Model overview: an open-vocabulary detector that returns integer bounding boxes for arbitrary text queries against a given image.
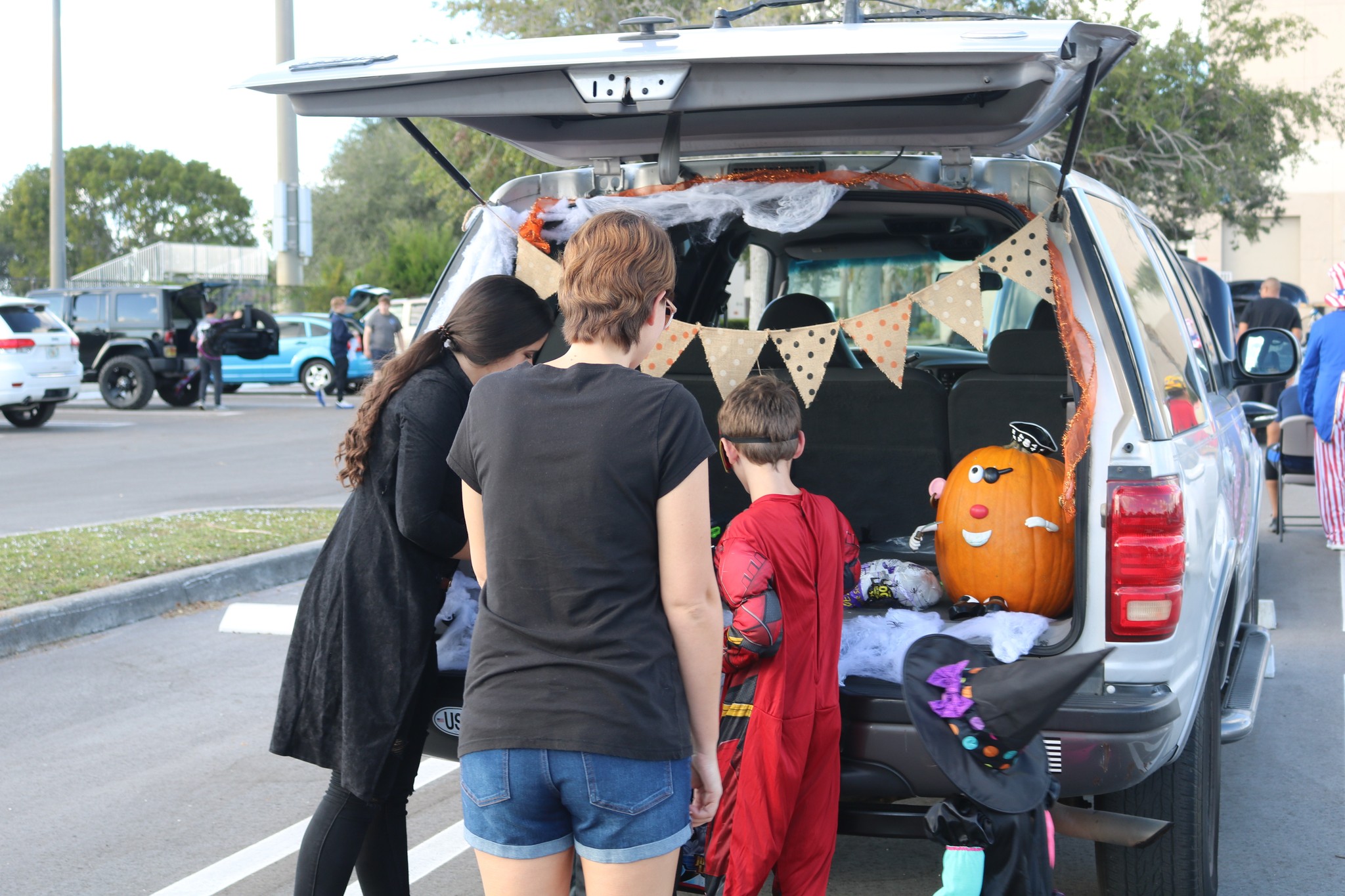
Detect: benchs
[635,335,946,543]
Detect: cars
[0,295,87,431]
[1225,280,1309,328]
[208,283,395,395]
[360,296,431,355]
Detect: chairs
[1026,298,1058,330]
[948,330,1067,469]
[753,293,863,368]
[1276,414,1323,543]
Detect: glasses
[661,297,675,326]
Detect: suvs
[240,1,1301,895]
[19,285,217,413]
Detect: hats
[902,633,1119,814]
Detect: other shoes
[336,399,353,408]
[1268,517,1285,531]
[318,387,326,406]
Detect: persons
[318,295,404,409]
[1218,413,1250,544]
[703,373,861,896]
[268,275,553,896]
[902,633,1116,896]
[1164,372,1199,433]
[446,209,724,896]
[1235,260,1345,552]
[189,303,242,410]
